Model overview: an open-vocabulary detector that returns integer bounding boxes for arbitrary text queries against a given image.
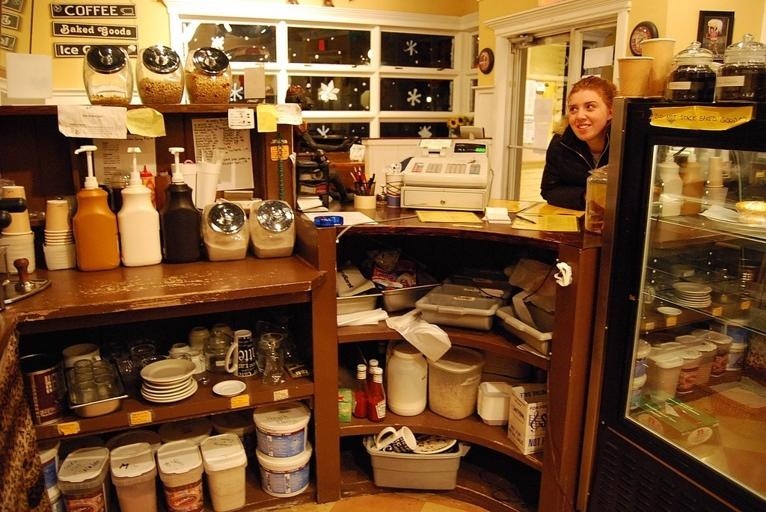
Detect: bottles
[351,356,387,422]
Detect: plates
[211,380,246,396]
[138,358,198,404]
[415,434,456,454]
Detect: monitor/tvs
[460,125,485,139]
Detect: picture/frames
[697,10,735,63]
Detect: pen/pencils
[350,165,376,196]
[516,214,537,224]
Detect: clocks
[629,21,658,56]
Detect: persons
[540,75,617,211]
[708,27,718,56]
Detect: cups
[703,148,735,208]
[638,38,676,99]
[259,333,287,385]
[374,424,418,452]
[64,343,100,367]
[72,359,119,404]
[670,280,713,309]
[43,200,77,272]
[385,173,404,207]
[119,321,231,375]
[223,330,258,378]
[0,186,36,275]
[617,54,653,96]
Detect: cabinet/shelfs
[0,103,325,512]
[296,201,597,512]
